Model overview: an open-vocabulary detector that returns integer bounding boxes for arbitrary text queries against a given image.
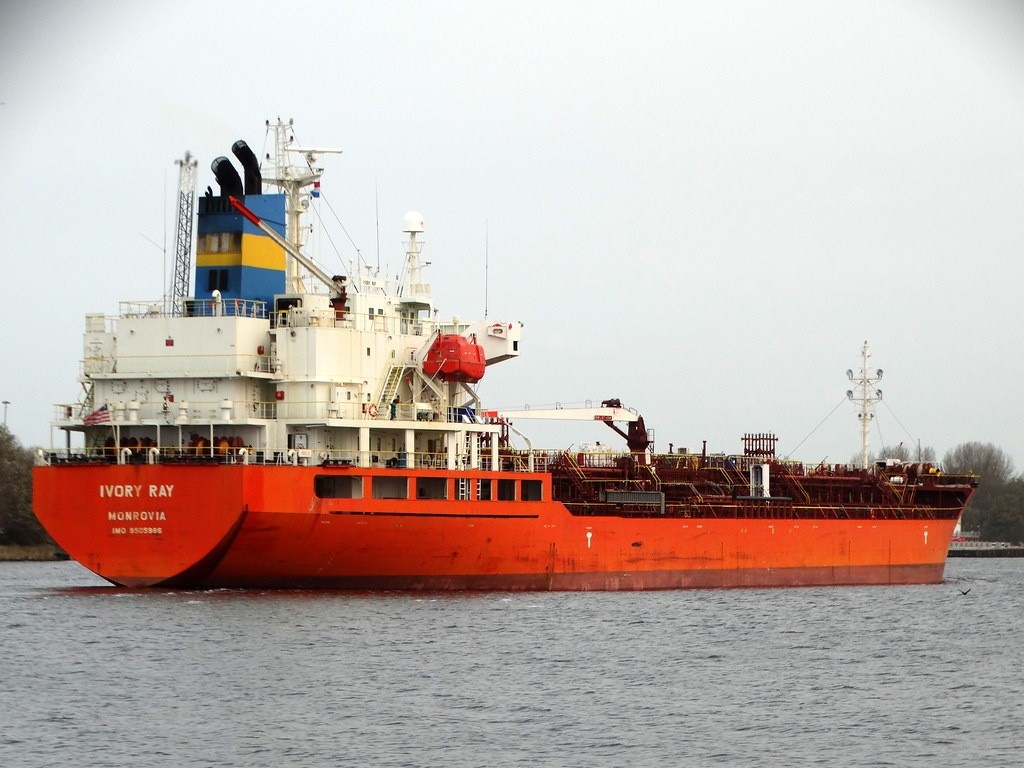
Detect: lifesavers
[369,405,378,417]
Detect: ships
[30,116,980,590]
